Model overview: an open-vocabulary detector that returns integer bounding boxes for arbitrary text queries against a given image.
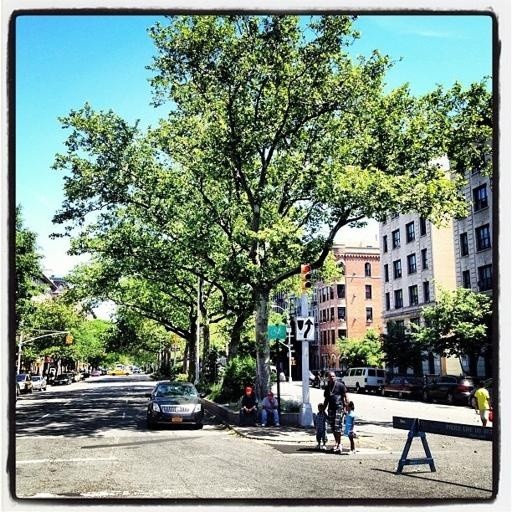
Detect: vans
[269,366,286,381]
[309,367,387,393]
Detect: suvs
[422,377,479,404]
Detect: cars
[144,381,211,430]
[391,375,421,386]
[52,369,100,385]
[107,362,140,376]
[468,378,492,408]
[16,374,47,398]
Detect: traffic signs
[170,348,180,351]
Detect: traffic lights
[300,265,311,291]
[66,335,73,344]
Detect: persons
[474,380,492,426]
[343,401,356,453]
[323,371,349,453]
[313,403,328,450]
[261,391,280,427]
[312,368,322,388]
[238,386,259,427]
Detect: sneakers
[316,445,357,455]
[239,423,282,427]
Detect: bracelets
[345,398,349,399]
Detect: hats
[245,386,252,393]
